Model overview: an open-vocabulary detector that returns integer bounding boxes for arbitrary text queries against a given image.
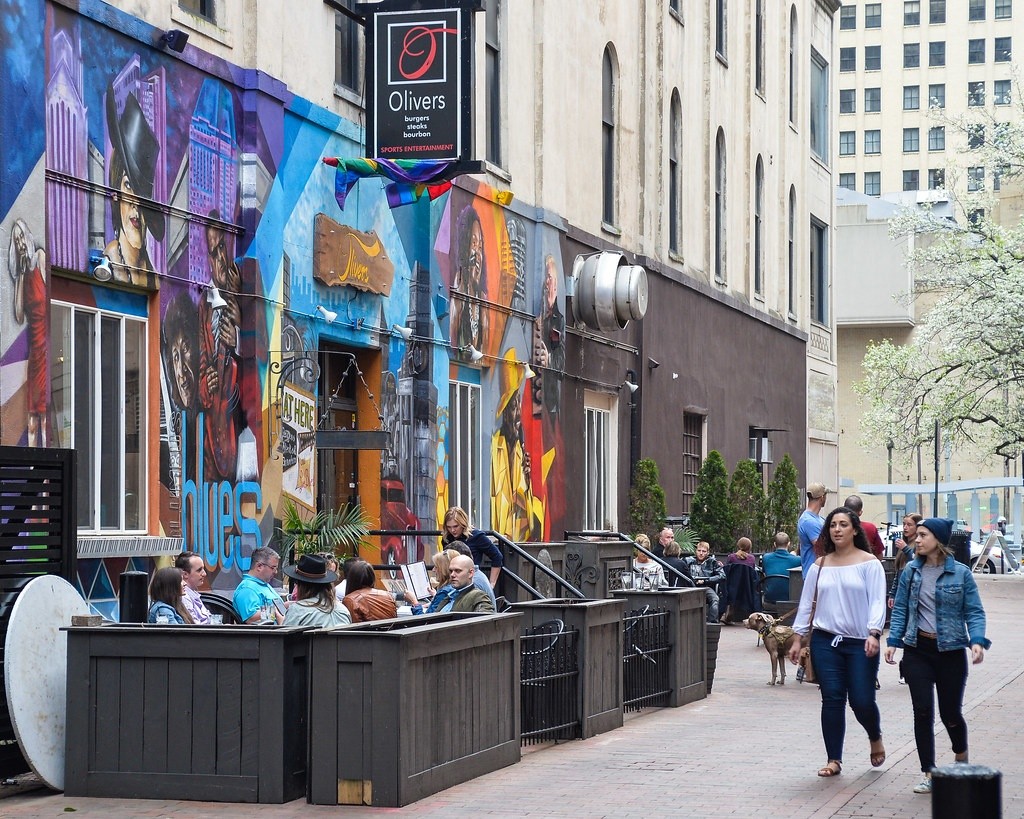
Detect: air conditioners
[748,437,774,464]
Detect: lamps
[314,304,338,321]
[392,322,414,341]
[90,256,113,280]
[518,361,537,379]
[619,379,640,394]
[164,28,190,53]
[498,190,515,206]
[462,342,483,362]
[208,288,228,311]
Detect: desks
[690,574,709,590]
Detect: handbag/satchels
[796,645,820,684]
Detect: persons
[797,484,831,684]
[844,495,884,690]
[440,506,504,590]
[789,506,888,777]
[884,518,993,793]
[888,513,924,684]
[762,532,801,603]
[147,567,195,624]
[403,540,496,615]
[174,551,214,625]
[232,546,293,626]
[335,557,360,601]
[652,528,697,587]
[342,561,398,623]
[632,534,669,587]
[275,552,352,628]
[682,541,727,622]
[727,537,755,569]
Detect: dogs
[743,612,812,686]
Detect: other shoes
[720,613,734,625]
[898,677,905,683]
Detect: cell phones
[273,598,287,616]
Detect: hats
[915,518,954,547]
[806,481,838,499]
[104,82,166,238]
[495,345,525,421]
[281,554,341,584]
[445,540,474,560]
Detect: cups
[267,605,275,622]
[690,564,698,578]
[621,572,632,591]
[210,614,223,625]
[261,605,268,625]
[634,572,645,591]
[649,573,659,592]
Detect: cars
[879,525,1019,575]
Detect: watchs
[869,632,880,640]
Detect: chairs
[725,563,758,619]
[709,570,722,595]
[620,605,650,652]
[756,575,790,647]
[519,618,564,689]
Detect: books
[399,560,433,599]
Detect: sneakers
[955,745,968,763]
[912,776,933,794]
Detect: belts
[918,630,938,639]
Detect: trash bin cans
[948,531,974,568]
[891,531,903,557]
[880,557,897,626]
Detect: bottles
[156,614,168,624]
[759,554,763,571]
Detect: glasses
[257,563,279,572]
[207,236,226,258]
[443,548,449,563]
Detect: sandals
[817,760,842,777]
[870,733,886,767]
